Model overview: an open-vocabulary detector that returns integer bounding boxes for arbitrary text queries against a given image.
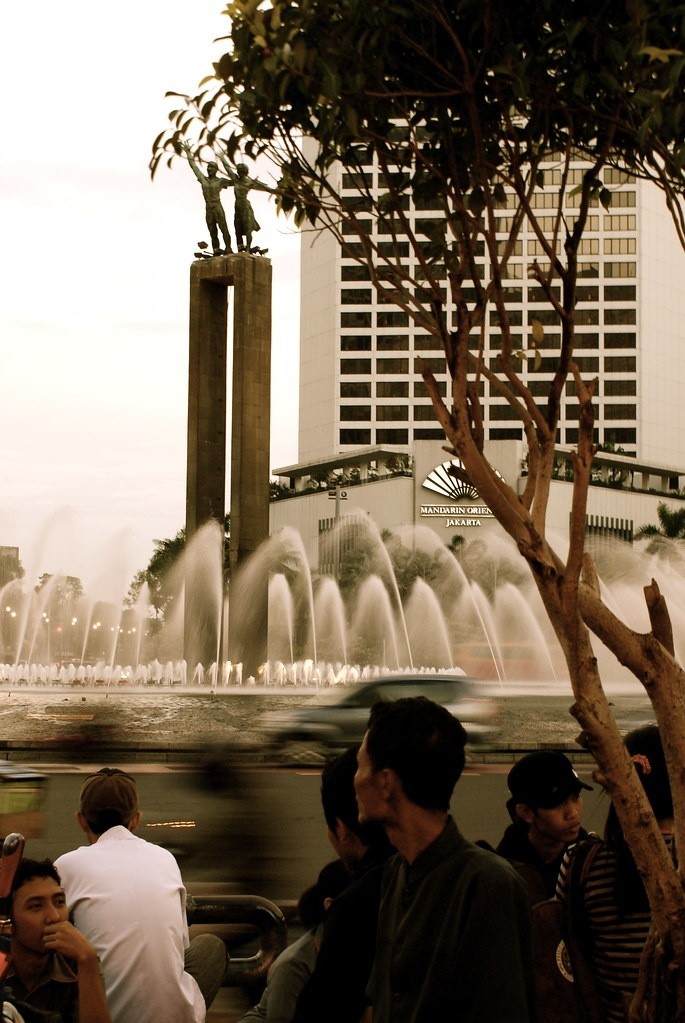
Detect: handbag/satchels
[526,841,601,1023]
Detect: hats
[79,768,138,826]
[507,751,594,809]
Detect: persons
[0,697,678,1023]
[182,140,235,256]
[216,150,276,251]
